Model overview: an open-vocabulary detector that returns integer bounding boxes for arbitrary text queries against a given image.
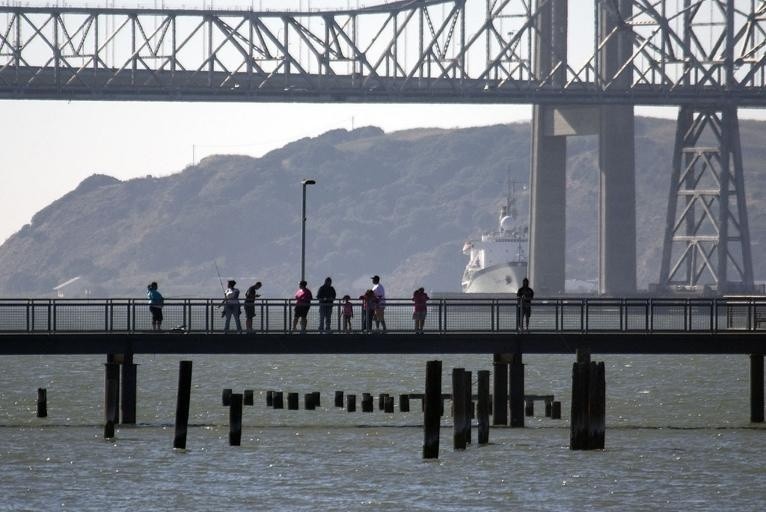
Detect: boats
[459,164,530,294]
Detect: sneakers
[373,329,387,333]
[416,330,424,334]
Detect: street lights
[298,178,318,283]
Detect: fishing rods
[215,261,229,304]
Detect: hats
[229,281,236,286]
[371,276,379,279]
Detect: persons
[411,289,430,335]
[369,274,387,333]
[146,280,164,331]
[242,281,262,334]
[418,287,428,317]
[317,276,337,335]
[515,278,534,335]
[288,281,312,335]
[359,289,380,333]
[216,279,245,334]
[338,294,354,332]
